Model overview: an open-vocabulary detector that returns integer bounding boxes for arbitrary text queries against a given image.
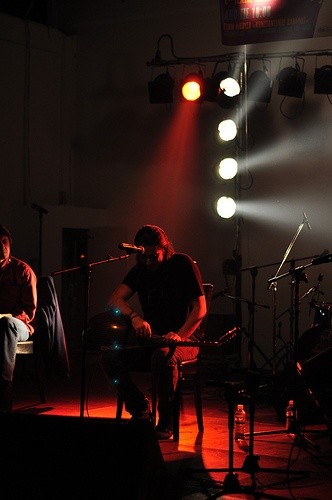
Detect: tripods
[189,218,332,500]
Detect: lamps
[148,53,332,106]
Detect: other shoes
[154,422,173,439]
[131,408,153,424]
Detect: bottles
[285,400,296,429]
[234,404,246,441]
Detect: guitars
[81,312,242,355]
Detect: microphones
[118,243,146,256]
[316,273,324,289]
[303,212,312,230]
[31,204,49,214]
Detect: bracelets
[128,310,135,317]
[130,314,139,320]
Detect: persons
[101,224,209,438]
[0,226,37,411]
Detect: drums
[312,304,332,327]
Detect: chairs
[116,283,213,442]
[15,276,70,403]
